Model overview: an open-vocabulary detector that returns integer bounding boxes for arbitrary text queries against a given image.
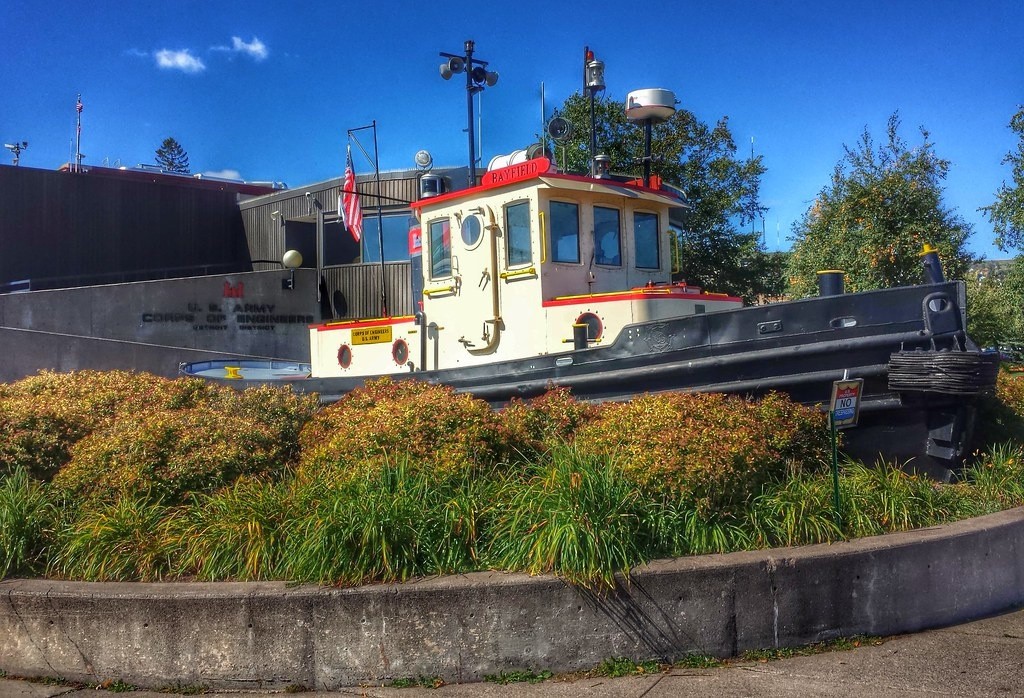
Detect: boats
[176,48,1006,482]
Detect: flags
[341,148,363,243]
